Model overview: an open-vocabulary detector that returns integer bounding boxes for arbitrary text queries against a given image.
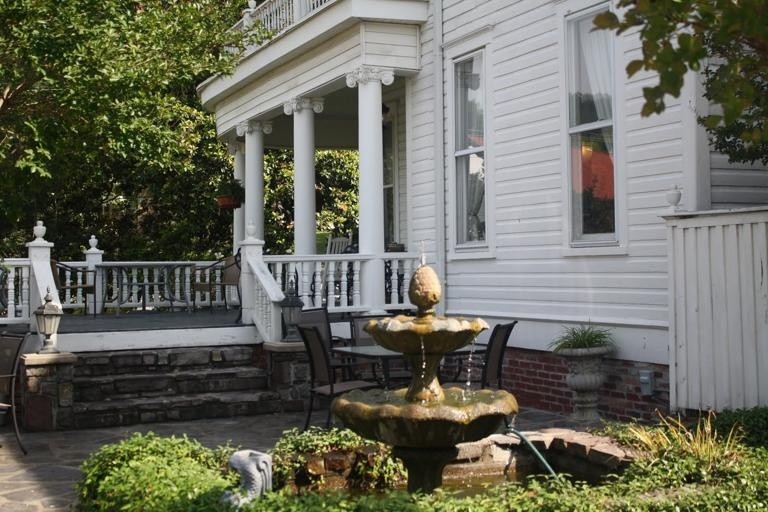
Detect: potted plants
[552,324,615,421]
[211,168,246,209]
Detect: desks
[90,260,197,317]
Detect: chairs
[316,229,353,283]
[294,307,517,431]
[191,246,242,315]
[48,259,99,320]
[0,330,32,458]
